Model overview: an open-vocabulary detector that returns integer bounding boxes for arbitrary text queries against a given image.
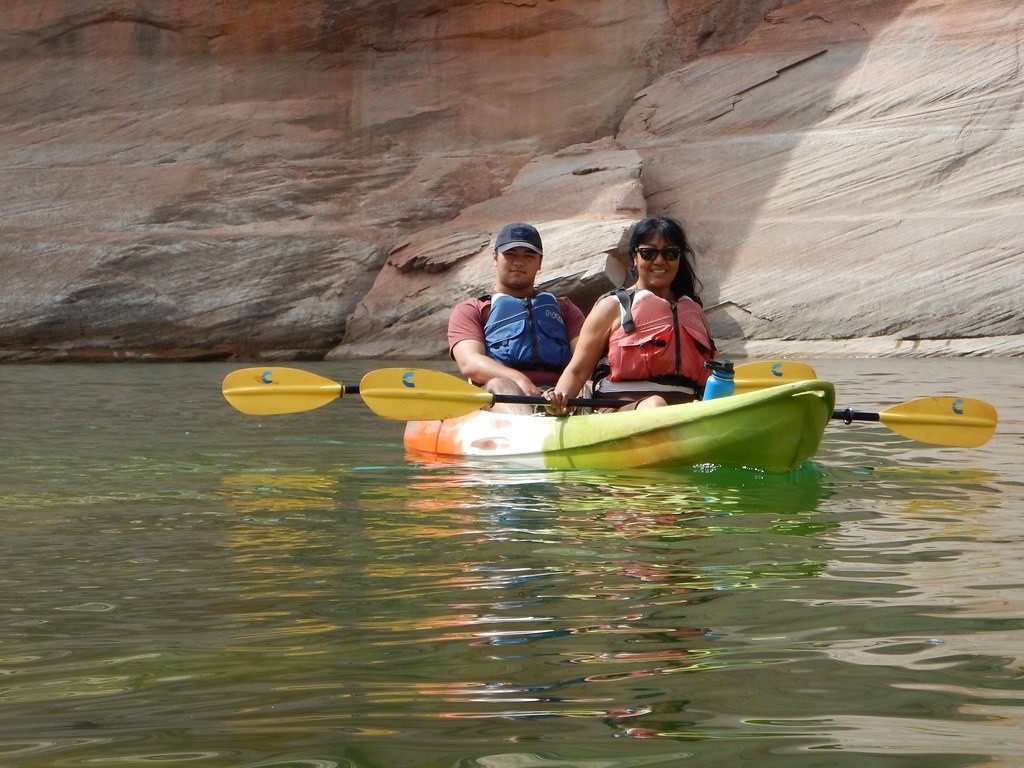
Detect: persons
[542,217,718,423]
[448,223,587,416]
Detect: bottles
[702,359,735,405]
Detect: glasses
[634,246,682,262]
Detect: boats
[403,379,835,475]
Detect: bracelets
[544,387,567,415]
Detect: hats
[493,222,543,255]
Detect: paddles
[222,365,999,451]
